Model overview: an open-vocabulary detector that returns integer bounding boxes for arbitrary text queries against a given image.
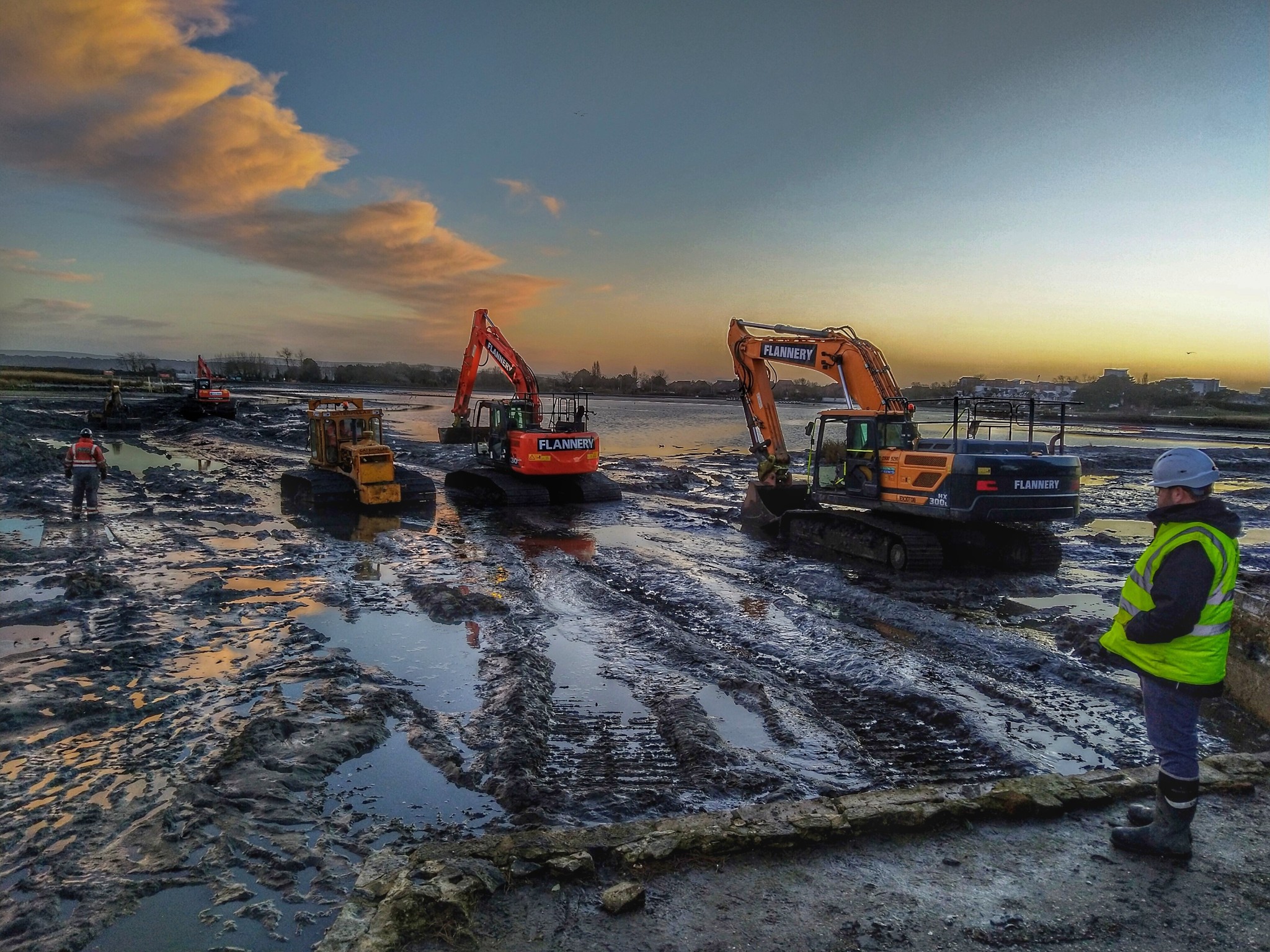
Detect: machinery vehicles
[91,385,142,431]
[281,399,436,517]
[190,355,237,420]
[726,315,1082,574]
[437,308,622,509]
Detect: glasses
[1154,487,1164,494]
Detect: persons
[329,420,353,445]
[1099,446,1241,859]
[64,428,107,519]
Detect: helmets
[1147,447,1219,488]
[80,428,93,435]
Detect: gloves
[65,469,72,479]
[99,467,108,480]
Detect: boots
[1126,803,1192,843]
[1109,766,1200,860]
